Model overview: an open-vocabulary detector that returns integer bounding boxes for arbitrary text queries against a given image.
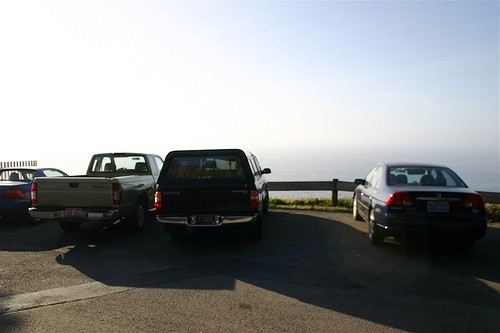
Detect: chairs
[397,174,434,185]
[105,163,116,172]
[136,162,147,172]
[9,173,19,181]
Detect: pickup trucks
[28,152,162,234]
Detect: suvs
[154,148,270,234]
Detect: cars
[351,162,487,248]
[0,166,69,226]
